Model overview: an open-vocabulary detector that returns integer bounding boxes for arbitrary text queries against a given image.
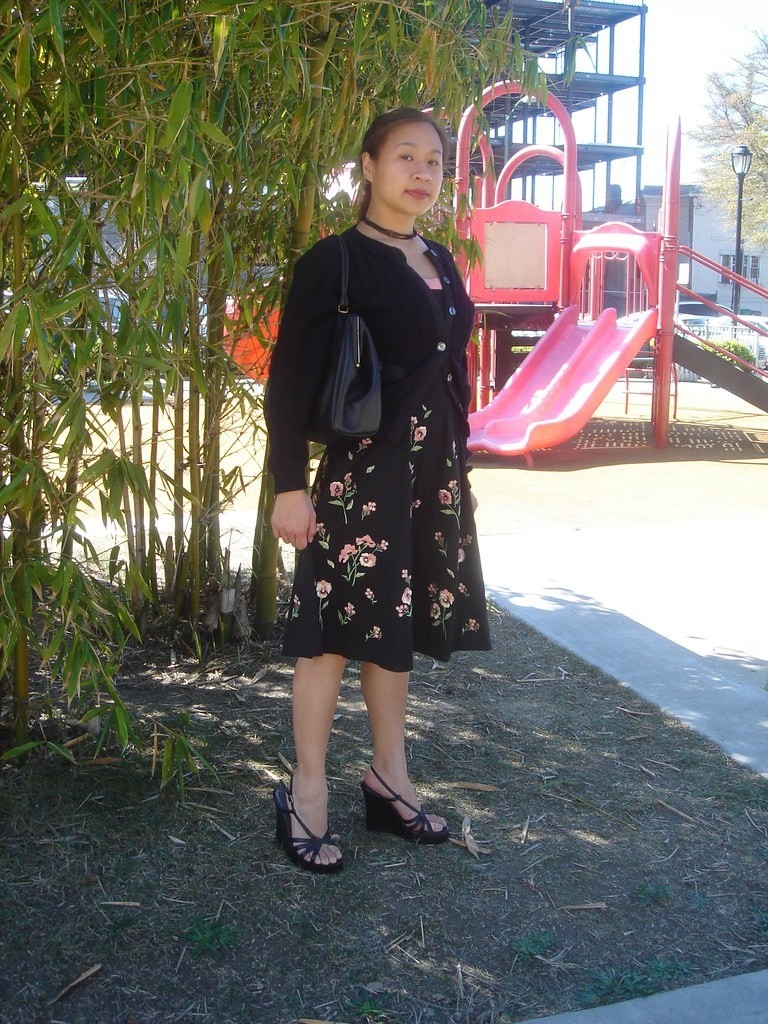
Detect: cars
[0,285,215,382]
[615,297,768,371]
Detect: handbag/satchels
[307,234,381,444]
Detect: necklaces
[363,217,418,238]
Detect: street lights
[732,142,754,336]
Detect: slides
[465,304,658,457]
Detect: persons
[263,109,495,875]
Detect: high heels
[361,766,449,844]
[273,775,343,872]
